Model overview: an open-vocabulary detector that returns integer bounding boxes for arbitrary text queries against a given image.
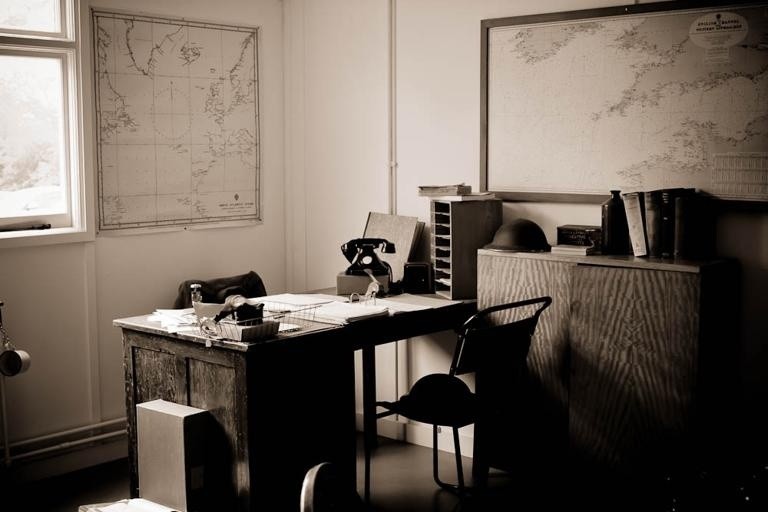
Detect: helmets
[485,217,552,254]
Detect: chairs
[361,296,556,502]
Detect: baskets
[200,299,330,339]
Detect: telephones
[341,239,395,275]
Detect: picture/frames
[477,0,768,214]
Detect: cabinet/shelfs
[472,244,725,510]
[429,199,504,301]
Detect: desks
[112,288,477,511]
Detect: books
[151,288,463,341]
[419,191,496,202]
[620,187,719,266]
[416,183,472,195]
[551,244,595,252]
[550,247,596,256]
